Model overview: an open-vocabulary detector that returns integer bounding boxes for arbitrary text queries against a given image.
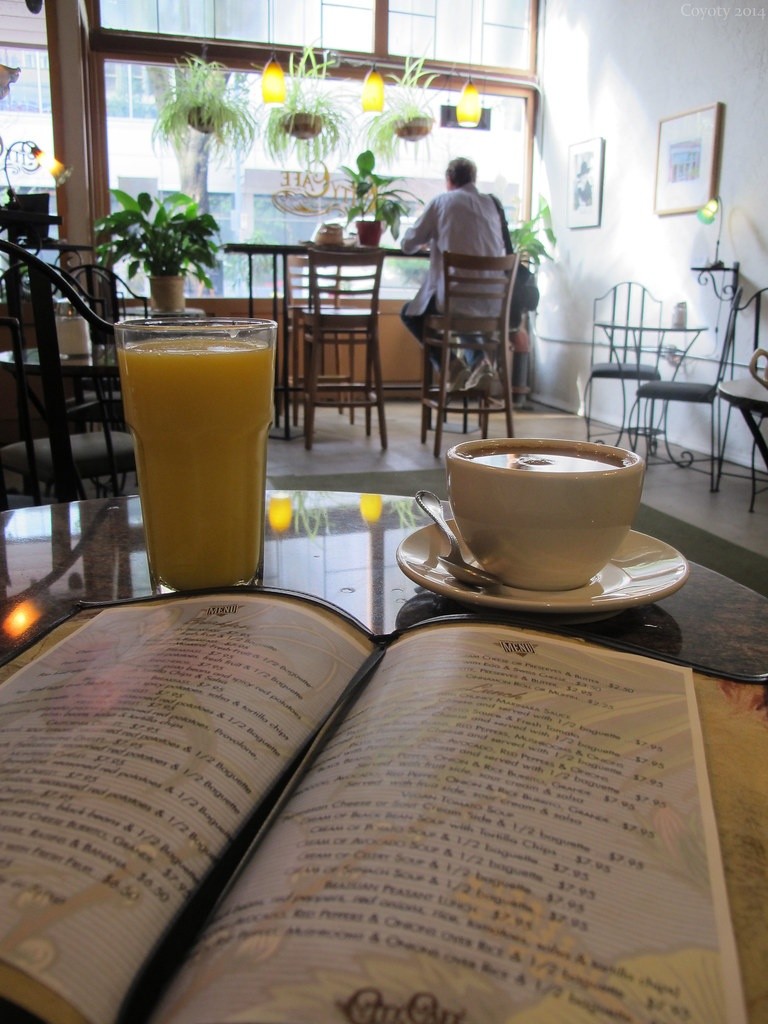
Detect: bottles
[55,297,93,358]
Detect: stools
[282,240,521,459]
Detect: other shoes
[472,364,494,392]
[446,359,472,393]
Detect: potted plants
[152,33,442,160]
[93,188,228,313]
[337,149,426,246]
[495,193,558,404]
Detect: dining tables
[0,490,768,682]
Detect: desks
[0,344,139,497]
[223,244,431,408]
[718,377,768,514]
[120,305,206,319]
[594,321,709,469]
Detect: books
[0,589,752,1024]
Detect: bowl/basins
[446,439,645,592]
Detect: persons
[577,162,591,207]
[400,157,511,407]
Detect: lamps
[362,0,385,116]
[456,0,482,128]
[695,195,724,268]
[261,0,286,108]
[4,140,74,214]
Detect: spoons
[416,490,499,586]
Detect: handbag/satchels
[504,265,540,311]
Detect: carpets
[267,466,767,601]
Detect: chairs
[0,238,148,511]
[714,287,768,513]
[634,286,744,493]
[582,281,662,463]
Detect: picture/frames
[653,102,723,215]
[566,137,605,228]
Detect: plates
[395,518,689,625]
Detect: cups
[671,301,686,329]
[112,316,278,596]
